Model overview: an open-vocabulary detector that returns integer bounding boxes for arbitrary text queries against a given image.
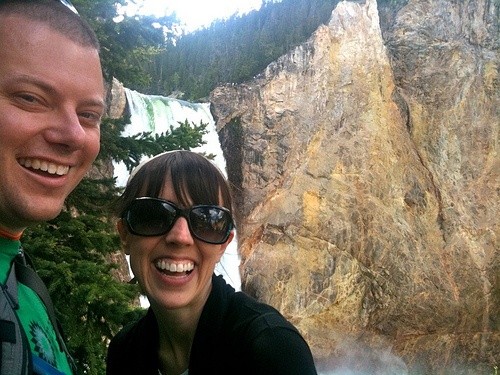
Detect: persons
[106,150,318,375]
[0,0,105,375]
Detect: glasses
[119,197,234,244]
